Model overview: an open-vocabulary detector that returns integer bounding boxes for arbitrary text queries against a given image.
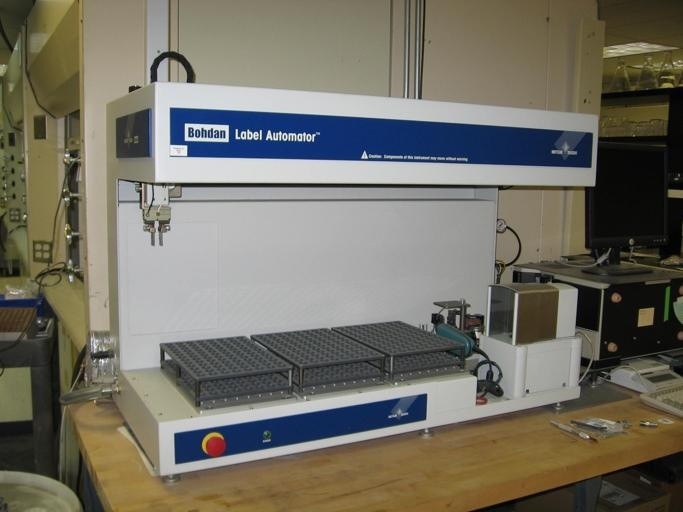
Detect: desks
[64,380,681,511]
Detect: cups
[599,115,667,137]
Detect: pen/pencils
[549,420,596,441]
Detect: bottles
[608,50,683,91]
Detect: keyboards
[638,382,683,418]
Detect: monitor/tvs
[583,141,667,275]
